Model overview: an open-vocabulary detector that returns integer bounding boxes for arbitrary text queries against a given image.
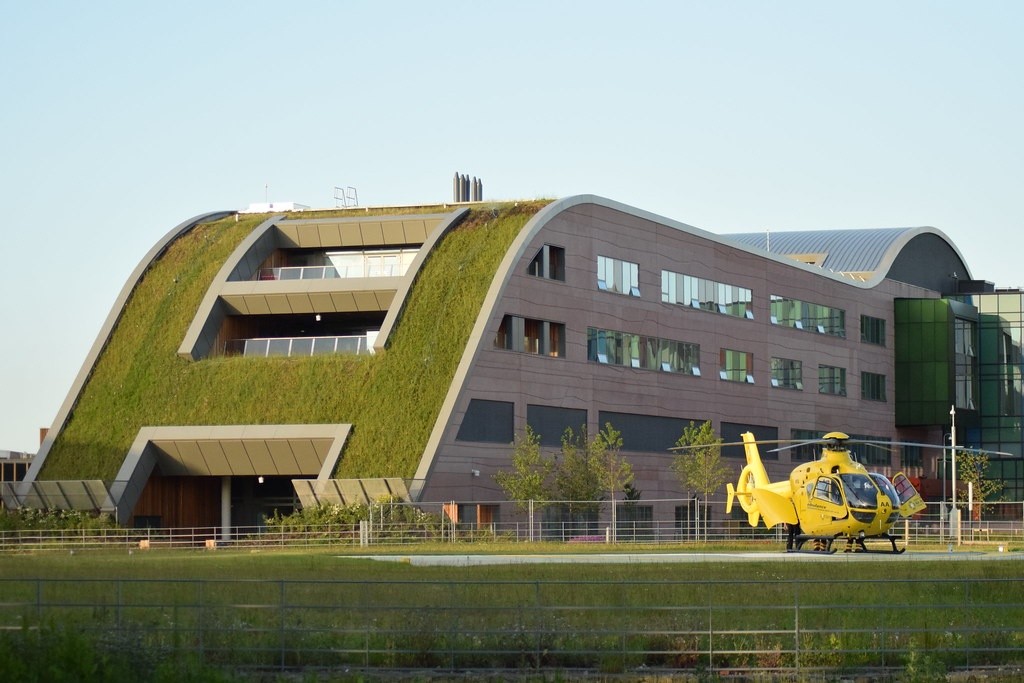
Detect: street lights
[949,404,962,546]
[942,433,952,520]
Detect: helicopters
[665,431,1012,555]
